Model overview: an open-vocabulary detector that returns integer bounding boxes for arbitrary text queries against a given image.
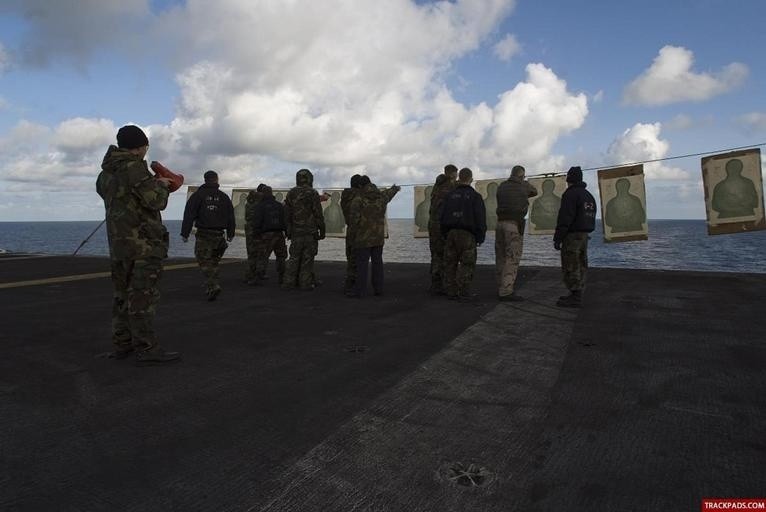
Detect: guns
[323,192,332,201]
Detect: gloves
[320,230,325,239]
[553,238,562,250]
[391,184,401,192]
[284,233,291,241]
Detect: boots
[556,290,585,308]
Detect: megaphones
[150,159,185,195]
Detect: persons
[94,124,184,362]
[423,164,539,303]
[552,166,597,307]
[182,170,236,302]
[244,170,401,298]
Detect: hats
[261,186,272,193]
[116,125,149,149]
[565,166,583,182]
[351,174,363,189]
[256,184,266,192]
[359,175,371,185]
[204,170,219,185]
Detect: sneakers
[239,273,475,304]
[205,285,222,302]
[498,293,525,302]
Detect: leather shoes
[134,348,185,367]
[111,346,136,360]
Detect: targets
[475,178,508,231]
[413,186,435,238]
[231,189,257,237]
[597,164,649,243]
[272,190,290,205]
[320,190,347,238]
[527,175,567,235]
[185,186,202,235]
[701,148,766,236]
